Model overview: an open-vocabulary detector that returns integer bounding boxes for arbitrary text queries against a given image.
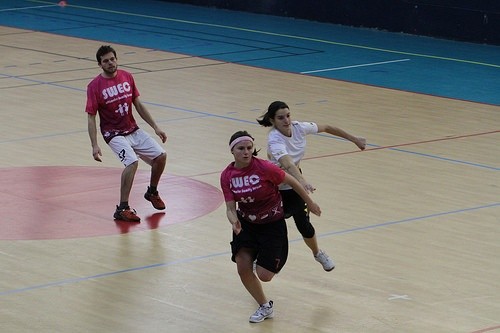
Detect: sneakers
[249,300,274,322]
[143,186,165,210]
[113,205,141,221]
[314,249,335,271]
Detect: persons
[86,46,167,223]
[257,101,366,271]
[221,131,321,323]
